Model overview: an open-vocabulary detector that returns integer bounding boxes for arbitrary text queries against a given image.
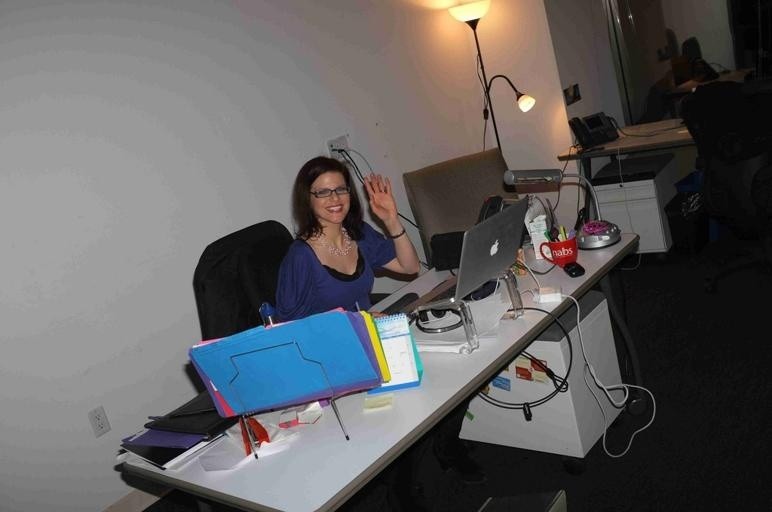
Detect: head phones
[415,309,467,334]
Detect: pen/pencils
[544,224,577,243]
[331,400,350,440]
[242,415,259,459]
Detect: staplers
[239,416,270,457]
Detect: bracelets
[390,229,410,238]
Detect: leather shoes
[383,476,427,512]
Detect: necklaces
[318,229,353,257]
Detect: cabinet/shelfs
[587,152,685,255]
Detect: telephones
[568,111,619,149]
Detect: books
[353,312,423,394]
[112,389,244,474]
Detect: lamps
[502,168,625,250]
[448,1,537,158]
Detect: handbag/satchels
[430,231,501,301]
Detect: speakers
[430,230,466,272]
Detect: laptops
[400,195,528,319]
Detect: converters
[538,286,562,303]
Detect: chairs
[193,218,296,340]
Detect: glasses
[311,187,350,198]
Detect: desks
[555,118,695,168]
[115,230,640,512]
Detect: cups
[539,235,578,267]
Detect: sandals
[439,455,488,485]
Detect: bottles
[258,301,280,329]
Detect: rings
[380,190,385,192]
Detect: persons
[267,151,423,322]
